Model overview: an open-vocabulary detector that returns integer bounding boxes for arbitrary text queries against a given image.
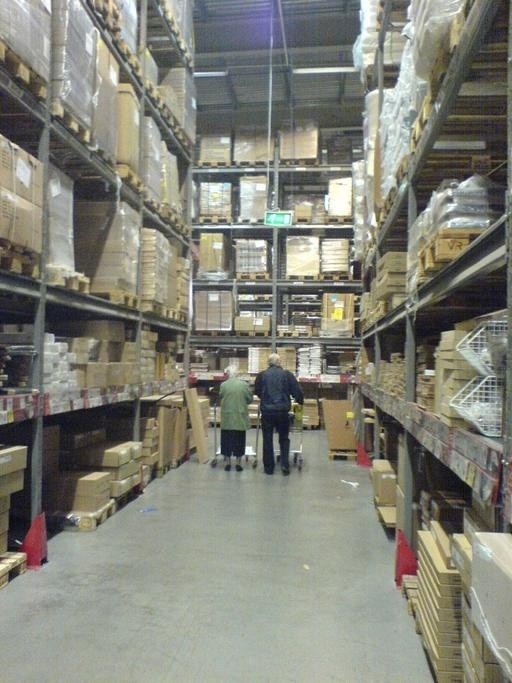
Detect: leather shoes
[225,464,230,471]
[236,465,242,471]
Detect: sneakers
[282,467,289,475]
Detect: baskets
[456,321,508,379]
[449,376,504,437]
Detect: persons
[253,353,305,476]
[216,363,253,472]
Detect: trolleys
[274,398,303,470]
[211,397,260,468]
